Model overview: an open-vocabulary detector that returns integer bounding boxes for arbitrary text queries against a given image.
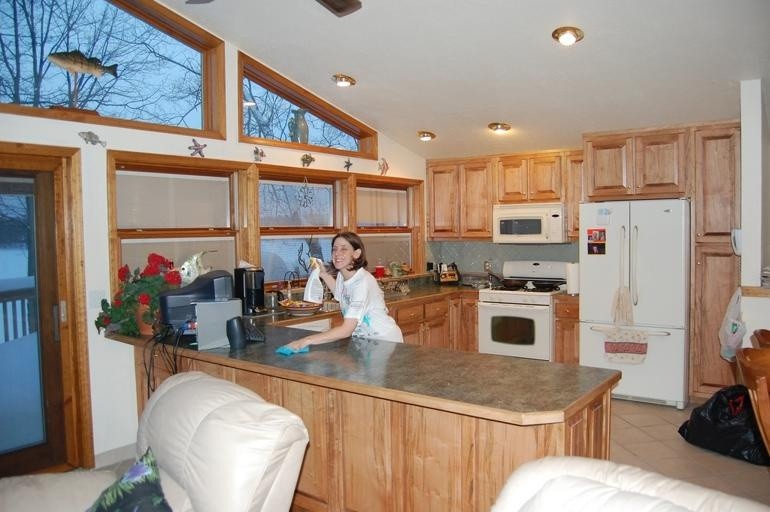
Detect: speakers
[226,316,247,349]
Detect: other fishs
[377,156,390,175]
[79,131,107,147]
[48,49,119,79]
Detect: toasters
[433,263,461,286]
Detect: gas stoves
[479,261,566,306]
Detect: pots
[488,272,528,288]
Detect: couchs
[488,443,765,511]
[1,370,311,511]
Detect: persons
[286,231,403,352]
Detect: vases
[288,108,308,145]
[135,298,164,337]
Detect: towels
[276,344,311,358]
[604,327,648,364]
[612,286,633,324]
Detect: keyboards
[243,316,265,342]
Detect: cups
[375,266,384,278]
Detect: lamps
[332,74,356,88]
[488,123,512,136]
[416,131,436,143]
[552,26,584,47]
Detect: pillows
[78,444,170,511]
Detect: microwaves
[491,202,571,244]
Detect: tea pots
[265,290,285,310]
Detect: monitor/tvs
[158,268,235,339]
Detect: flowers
[97,254,183,335]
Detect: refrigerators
[579,197,689,409]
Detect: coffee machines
[234,267,267,315]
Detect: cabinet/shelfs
[426,157,491,242]
[566,148,583,237]
[494,151,564,203]
[582,131,691,199]
[105,149,426,300]
[392,293,453,348]
[692,120,741,242]
[694,244,738,398]
[553,295,578,366]
[452,289,479,353]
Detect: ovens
[477,302,553,363]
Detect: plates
[281,302,323,316]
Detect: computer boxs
[190,297,243,351]
